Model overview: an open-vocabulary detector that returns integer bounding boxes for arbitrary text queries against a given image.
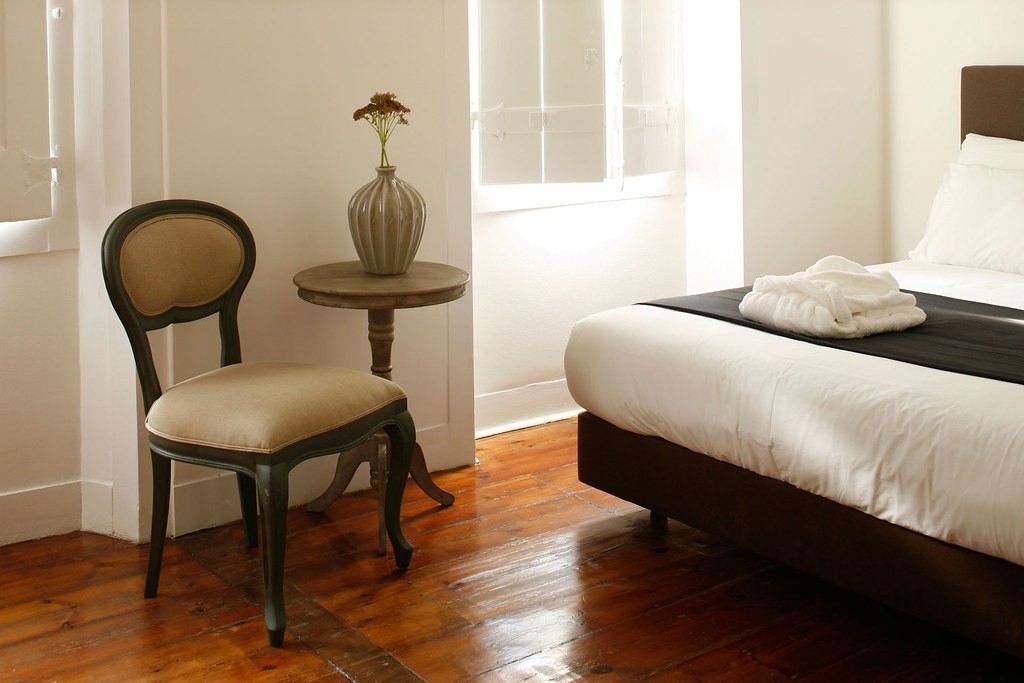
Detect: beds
[564,61,1024,665]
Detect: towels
[737,253,927,341]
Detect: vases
[344,168,430,277]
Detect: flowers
[353,91,414,172]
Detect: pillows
[924,165,1024,275]
[909,133,1019,270]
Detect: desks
[293,261,471,571]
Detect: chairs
[101,201,419,634]
